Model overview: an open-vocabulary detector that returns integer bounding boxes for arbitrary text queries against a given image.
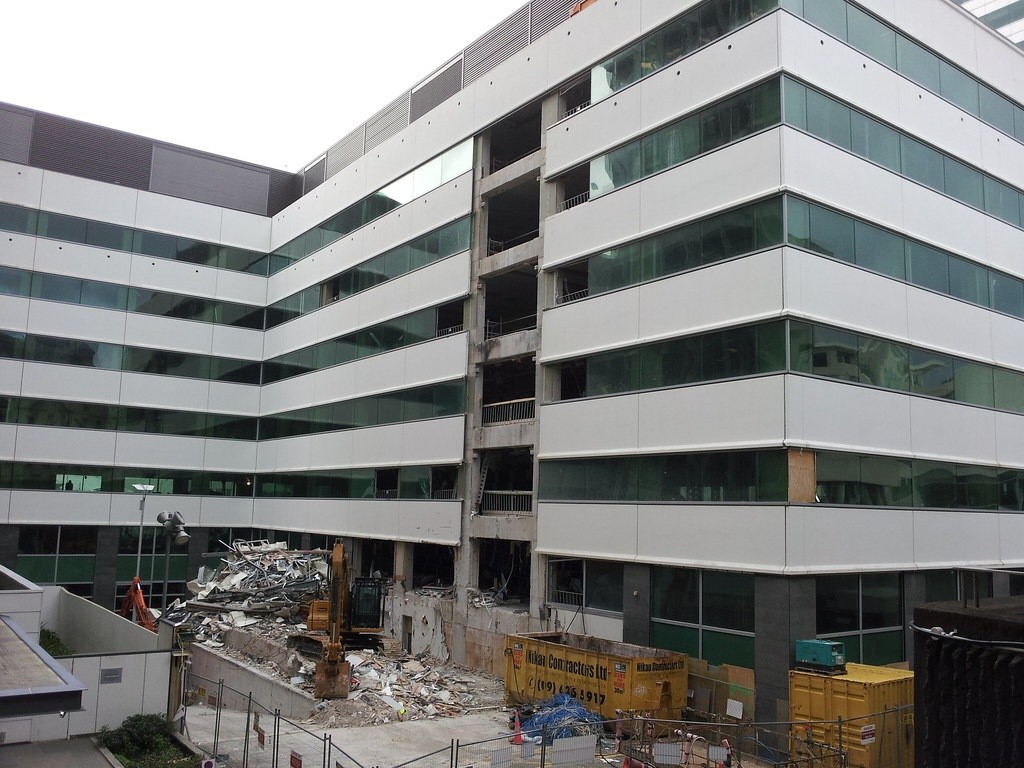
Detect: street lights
[156,511,191,618]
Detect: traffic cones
[509,711,524,744]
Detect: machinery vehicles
[117,576,157,632]
[287,537,400,698]
